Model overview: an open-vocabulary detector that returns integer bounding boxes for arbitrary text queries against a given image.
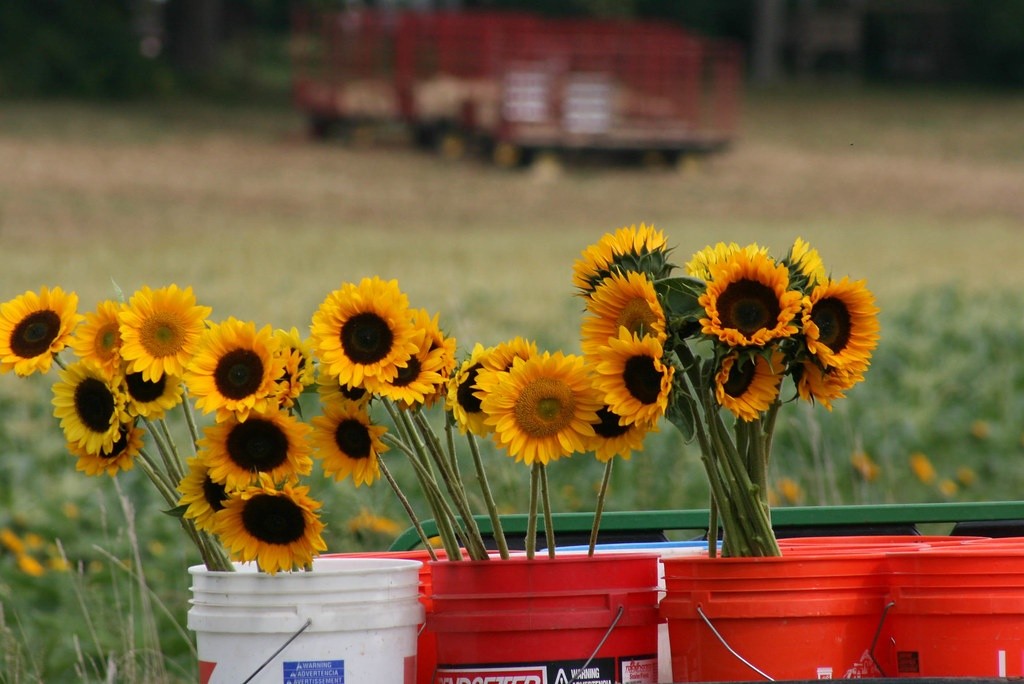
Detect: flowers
[1,221,885,578]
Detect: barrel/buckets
[183,535,1024,684]
